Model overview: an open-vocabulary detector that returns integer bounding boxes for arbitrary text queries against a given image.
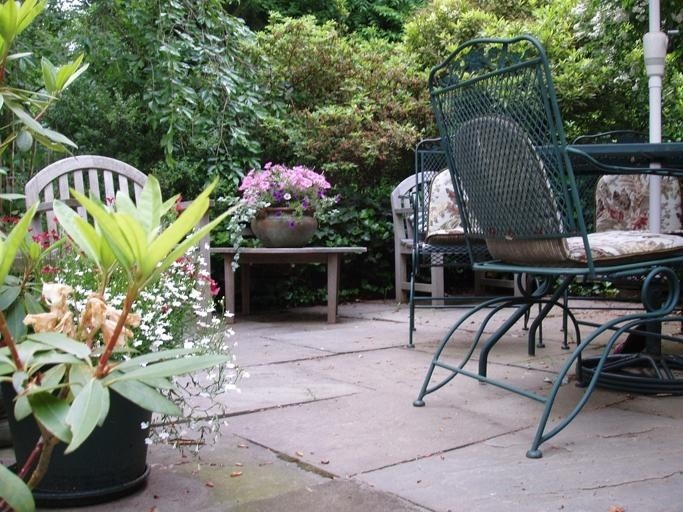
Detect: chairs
[404,133,546,357]
[25,155,215,323]
[558,131,683,351]
[391,167,527,307]
[412,36,683,459]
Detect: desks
[209,247,367,323]
[535,142,682,398]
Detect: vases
[0,364,154,507]
[250,208,318,248]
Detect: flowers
[0,199,251,470]
[218,162,341,272]
